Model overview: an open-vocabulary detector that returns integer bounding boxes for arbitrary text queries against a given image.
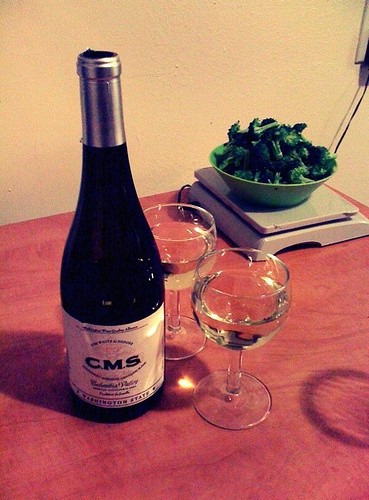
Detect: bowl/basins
[208,142,332,209]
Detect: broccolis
[216,118,336,183]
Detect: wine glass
[142,203,218,362]
[189,247,294,430]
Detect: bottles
[59,49,166,424]
